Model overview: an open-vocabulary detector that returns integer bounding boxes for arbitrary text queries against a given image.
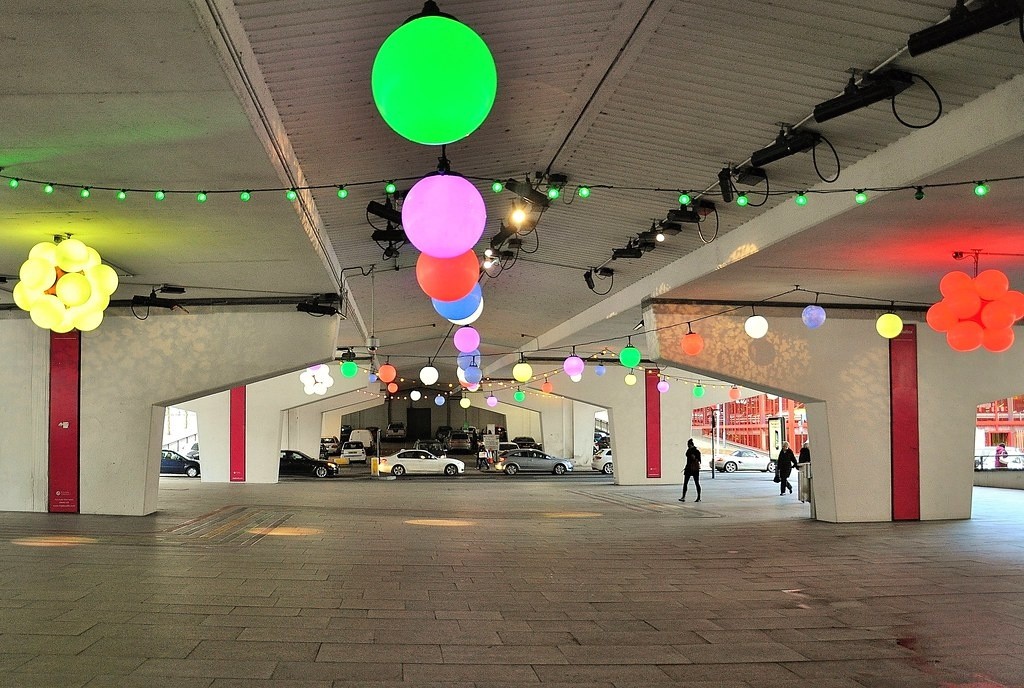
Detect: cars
[341,441,367,463]
[709,450,776,473]
[443,429,471,454]
[436,426,453,443]
[160,449,200,477]
[497,442,521,462]
[386,424,407,442]
[461,426,477,438]
[186,443,199,459]
[366,427,381,442]
[494,449,574,476]
[593,437,611,454]
[321,436,340,457]
[341,425,352,435]
[511,437,542,450]
[413,439,447,458]
[319,445,329,459]
[591,448,614,475]
[278,450,339,478]
[595,428,610,443]
[380,449,465,477]
[349,430,375,456]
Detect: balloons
[300,363,333,395]
[434,396,445,405]
[420,366,439,386]
[487,396,497,407]
[875,313,903,338]
[401,175,487,326]
[410,391,421,401]
[460,398,471,408]
[570,373,581,382]
[564,357,584,376]
[925,270,1024,353]
[619,347,641,368]
[656,381,669,392]
[681,333,706,356]
[378,364,396,383]
[542,382,552,393]
[513,363,532,382]
[369,374,377,383]
[745,315,769,339]
[594,366,604,376]
[387,383,398,393]
[371,15,498,145]
[625,374,636,385]
[453,327,483,392]
[514,391,525,402]
[13,239,119,334]
[802,305,826,329]
[340,361,358,378]
[729,388,741,399]
[693,386,704,397]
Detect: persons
[799,443,811,463]
[775,441,800,495]
[473,440,491,470]
[995,442,1008,467]
[678,438,701,502]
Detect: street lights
[711,408,720,478]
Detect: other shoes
[789,486,792,494]
[694,499,701,502]
[679,499,685,502]
[477,467,482,470]
[486,467,490,470]
[780,493,786,496]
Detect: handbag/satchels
[479,452,487,459]
[774,475,780,483]
